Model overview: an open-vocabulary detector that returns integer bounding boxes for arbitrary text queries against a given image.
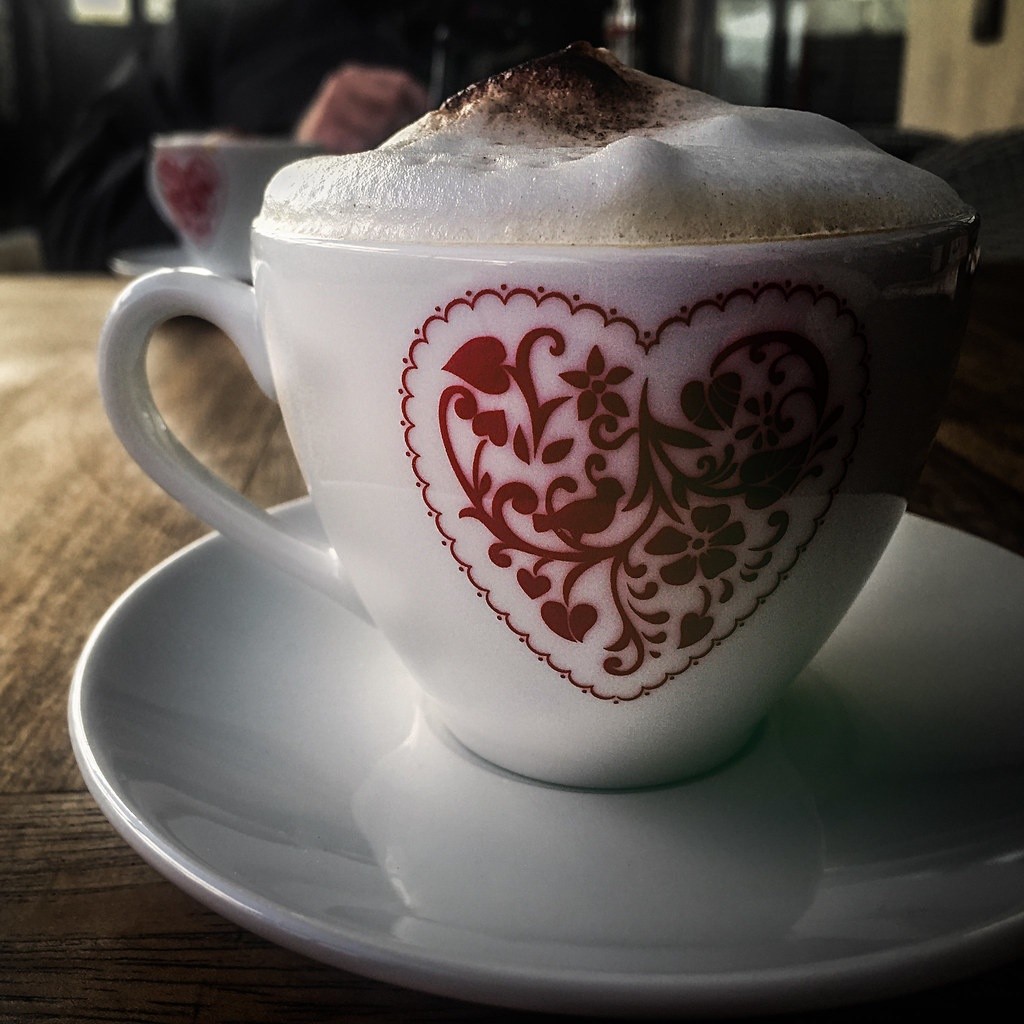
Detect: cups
[149,136,341,279]
[97,215,977,795]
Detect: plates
[108,247,196,278]
[65,490,1023,1009]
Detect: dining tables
[0,271,1024,1021]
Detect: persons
[34,0,613,256]
